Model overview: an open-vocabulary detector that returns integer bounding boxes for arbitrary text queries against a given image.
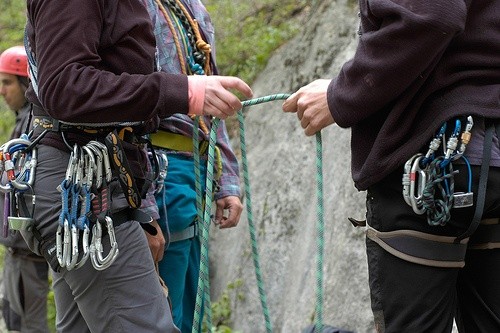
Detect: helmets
[0,45,29,77]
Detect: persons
[281,0,500,333]
[19,0,254,333]
[140,0,245,333]
[0,45,50,333]
